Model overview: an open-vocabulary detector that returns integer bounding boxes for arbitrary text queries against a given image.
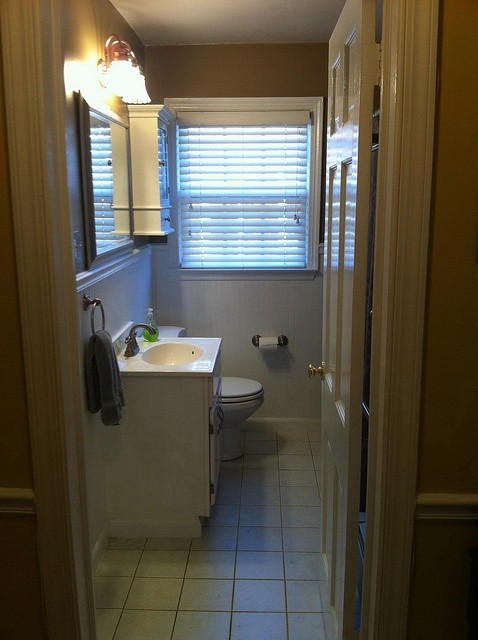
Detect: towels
[84,330,125,426]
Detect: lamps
[81,34,152,104]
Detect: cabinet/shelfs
[93,378,228,538]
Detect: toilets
[157,325,265,461]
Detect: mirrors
[75,89,150,270]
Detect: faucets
[123,323,157,357]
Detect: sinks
[141,342,204,366]
